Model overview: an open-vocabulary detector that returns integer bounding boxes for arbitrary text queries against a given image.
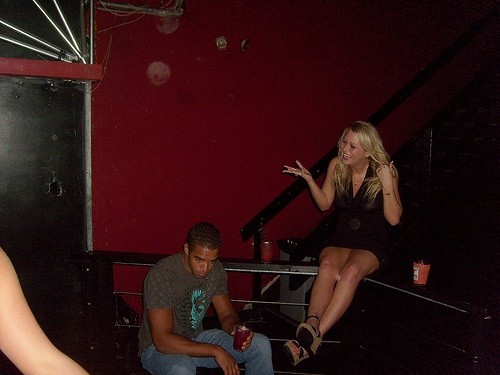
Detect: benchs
[80,251,500,375]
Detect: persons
[282,120,402,366]
[137,222,274,375]
[0,247,90,375]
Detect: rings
[378,168,381,171]
[295,174,297,176]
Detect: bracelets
[384,191,393,195]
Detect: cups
[413,257,431,285]
[232,322,252,353]
[260,241,274,264]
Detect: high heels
[284,339,310,366]
[296,322,322,356]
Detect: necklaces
[353,173,362,185]
